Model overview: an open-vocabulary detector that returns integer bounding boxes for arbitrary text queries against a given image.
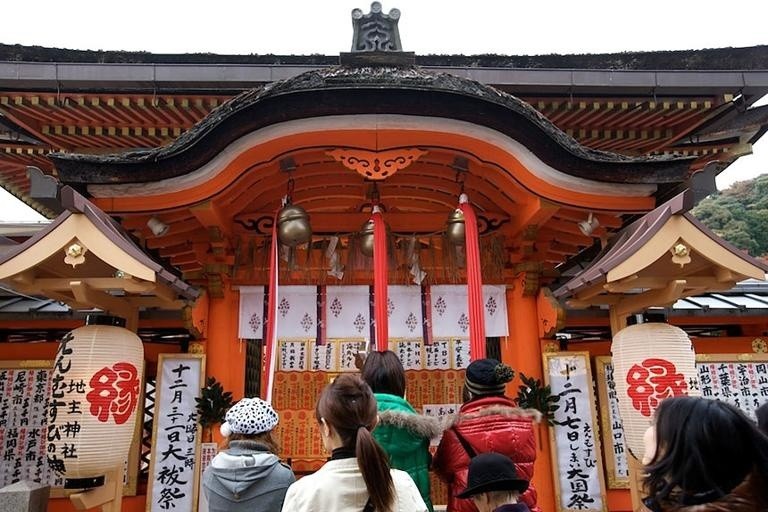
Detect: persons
[285,375,429,511]
[432,359,542,511]
[454,454,536,512]
[199,397,296,511]
[359,351,430,508]
[634,395,767,511]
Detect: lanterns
[47,326,144,487]
[609,323,697,464]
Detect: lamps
[578,217,600,237]
[147,218,169,237]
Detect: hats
[465,360,514,395]
[457,454,529,498]
[220,397,278,436]
